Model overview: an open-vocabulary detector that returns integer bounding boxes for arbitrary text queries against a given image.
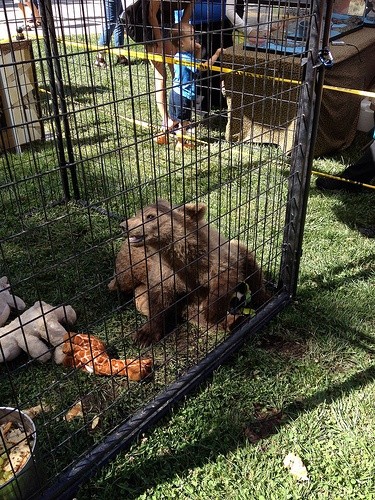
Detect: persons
[188,0,269,117]
[93,0,130,68]
[121,0,197,133]
[155,20,228,152]
[15,0,42,29]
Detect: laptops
[287,11,364,43]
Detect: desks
[216,19,375,145]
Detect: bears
[108,202,266,349]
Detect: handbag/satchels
[118,4,154,48]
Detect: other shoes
[115,54,130,65]
[154,131,168,145]
[19,24,31,31]
[175,134,195,148]
[94,56,108,68]
[30,21,42,28]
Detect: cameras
[332,40,345,46]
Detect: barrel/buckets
[0,406,41,500]
